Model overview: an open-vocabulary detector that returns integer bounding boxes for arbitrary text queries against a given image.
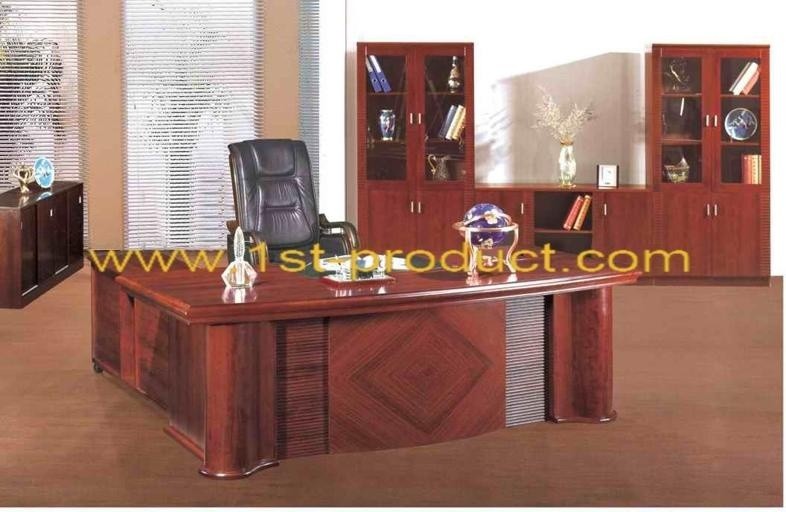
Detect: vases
[558,144,579,190]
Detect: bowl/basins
[664,164,690,184]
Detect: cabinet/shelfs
[0,178,83,309]
[644,42,772,287]
[474,182,652,286]
[356,40,475,267]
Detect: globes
[452,201,520,276]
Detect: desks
[82,243,646,480]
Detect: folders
[365,54,394,93]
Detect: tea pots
[427,153,451,181]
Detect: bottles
[220,224,259,292]
[448,55,461,95]
[222,287,261,306]
[379,109,396,141]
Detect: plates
[33,157,54,190]
[725,108,758,141]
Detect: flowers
[528,81,598,144]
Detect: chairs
[224,137,362,270]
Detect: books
[740,152,762,184]
[438,105,466,141]
[728,61,760,96]
[563,194,592,231]
